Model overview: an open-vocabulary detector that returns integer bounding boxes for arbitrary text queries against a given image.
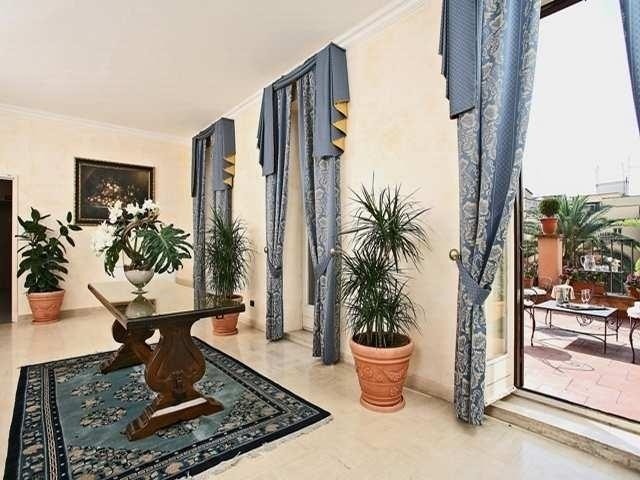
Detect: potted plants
[194,200,264,336]
[13,206,83,324]
[537,198,561,235]
[323,169,436,412]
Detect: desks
[85,281,248,442]
[529,300,619,354]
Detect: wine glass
[581,288,591,305]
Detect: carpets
[4,337,335,480]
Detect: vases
[124,294,156,320]
[121,264,156,294]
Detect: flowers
[89,197,196,279]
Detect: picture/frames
[73,155,156,227]
